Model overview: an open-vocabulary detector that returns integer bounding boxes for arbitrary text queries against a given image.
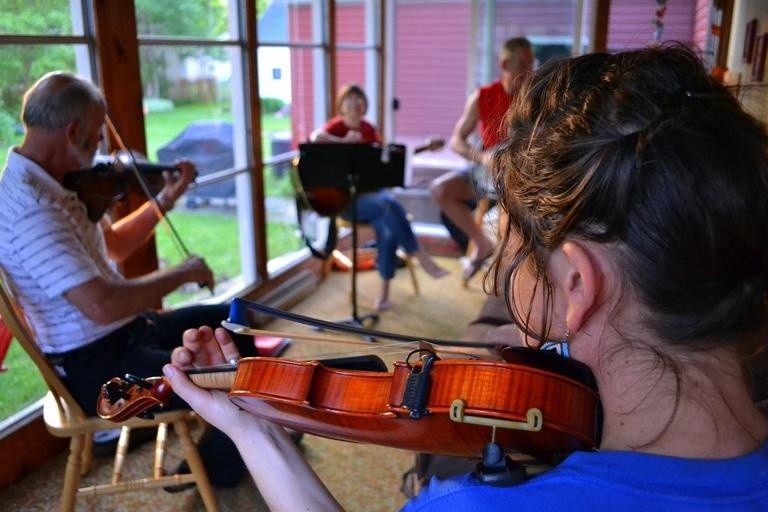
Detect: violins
[97,348,599,486]
[64,149,199,212]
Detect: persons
[160,42,767,510]
[430,35,535,276]
[307,84,451,312]
[0,71,305,491]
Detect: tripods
[309,192,381,347]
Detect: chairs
[0,264,219,512]
[292,157,420,303]
[462,192,504,289]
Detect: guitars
[291,137,445,218]
[475,140,507,197]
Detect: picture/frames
[741,19,768,81]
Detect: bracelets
[153,189,173,204]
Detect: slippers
[462,248,495,279]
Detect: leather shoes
[286,431,303,445]
[161,466,195,492]
